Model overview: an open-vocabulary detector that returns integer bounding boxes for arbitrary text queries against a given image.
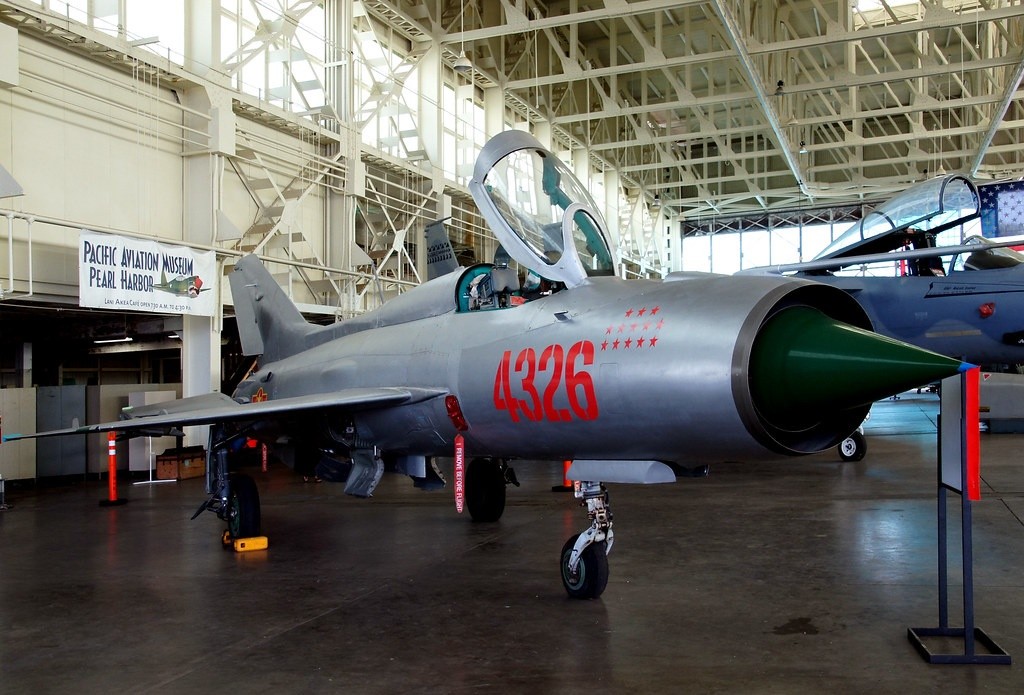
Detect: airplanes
[2,129,1022,603]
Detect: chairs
[491,267,521,308]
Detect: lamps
[774,80,785,96]
[936,97,947,176]
[797,180,807,191]
[694,176,703,238]
[167,331,180,339]
[91,329,137,346]
[652,130,663,206]
[453,0,471,71]
[799,140,807,154]
